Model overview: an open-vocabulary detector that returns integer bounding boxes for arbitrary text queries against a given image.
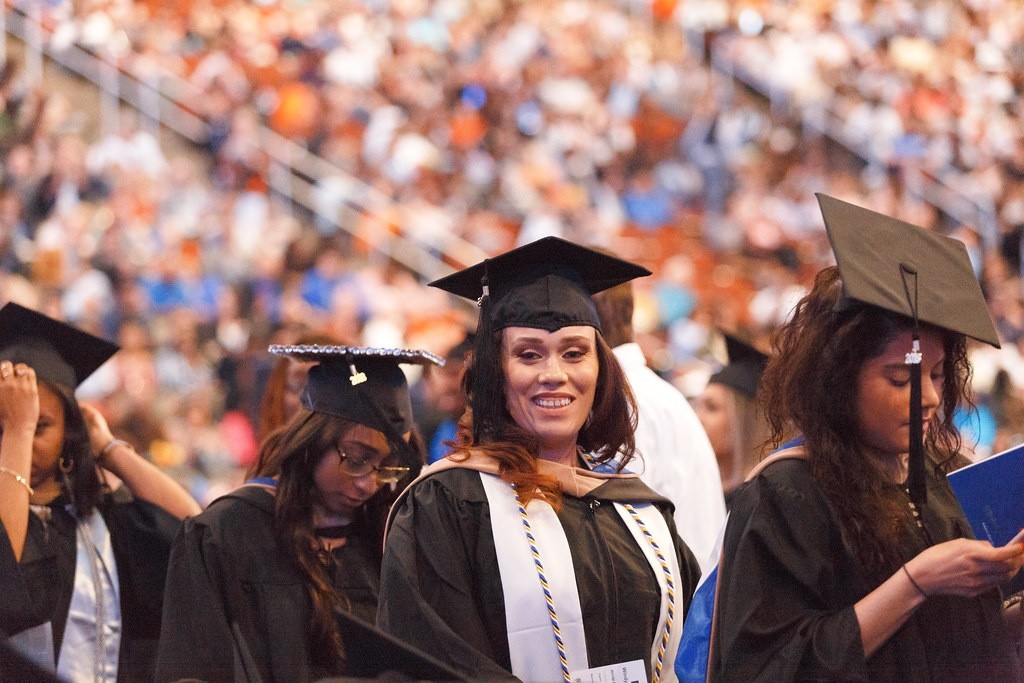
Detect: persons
[155,343,446,683]
[377,233,701,683]
[577,240,727,595]
[0,0,1024,514]
[692,323,790,511]
[713,192,1024,683]
[0,301,204,682]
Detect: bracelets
[902,563,933,604]
[96,440,136,469]
[0,466,34,497]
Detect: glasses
[332,440,413,486]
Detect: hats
[270,344,446,436]
[427,235,654,448]
[703,323,774,403]
[1,302,124,395]
[813,190,1004,511]
